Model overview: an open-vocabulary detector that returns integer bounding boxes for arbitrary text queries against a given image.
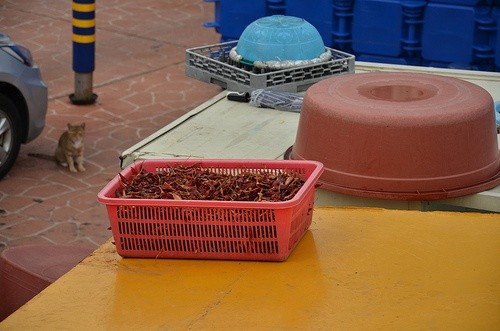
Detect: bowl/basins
[230,14,332,69]
[283,72,500,200]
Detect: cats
[26,122,86,173]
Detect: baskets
[97,160,324,262]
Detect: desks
[0,206,500,331]
[118,61,500,212]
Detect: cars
[0,31,49,182]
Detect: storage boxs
[185,39,356,97]
[97,159,325,261]
